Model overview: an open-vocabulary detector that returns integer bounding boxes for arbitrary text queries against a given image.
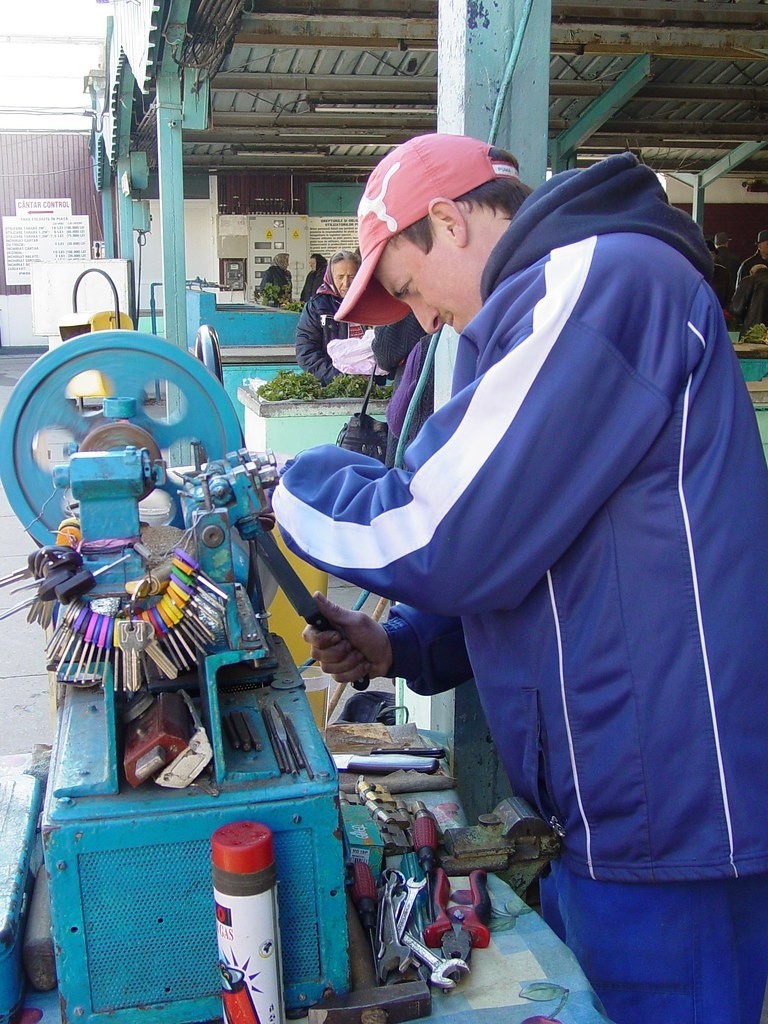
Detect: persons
[372,309,435,468]
[271,133,768,1024]
[300,254,328,302]
[704,230,768,330]
[260,253,293,305]
[295,250,388,388]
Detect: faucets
[227,282,233,289]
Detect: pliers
[436,870,497,968]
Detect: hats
[714,232,732,244]
[757,230,768,243]
[706,240,715,252]
[333,133,518,327]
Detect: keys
[190,773,219,797]
[0,545,230,692]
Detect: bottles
[211,821,286,1024]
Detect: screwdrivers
[413,814,437,926]
[352,859,384,985]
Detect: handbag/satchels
[337,365,388,465]
